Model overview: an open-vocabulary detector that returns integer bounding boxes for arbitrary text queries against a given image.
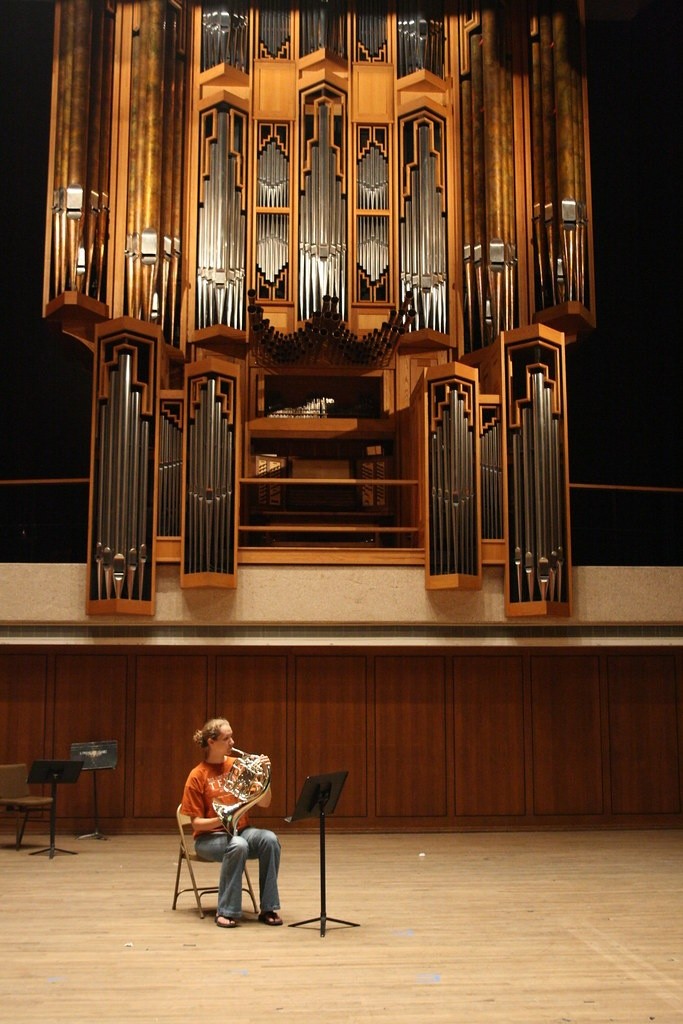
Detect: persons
[180,718,283,927]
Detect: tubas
[211,747,272,839]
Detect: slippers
[258,910,284,926]
[215,912,236,927]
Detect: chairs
[0,763,54,852]
[172,803,259,919]
